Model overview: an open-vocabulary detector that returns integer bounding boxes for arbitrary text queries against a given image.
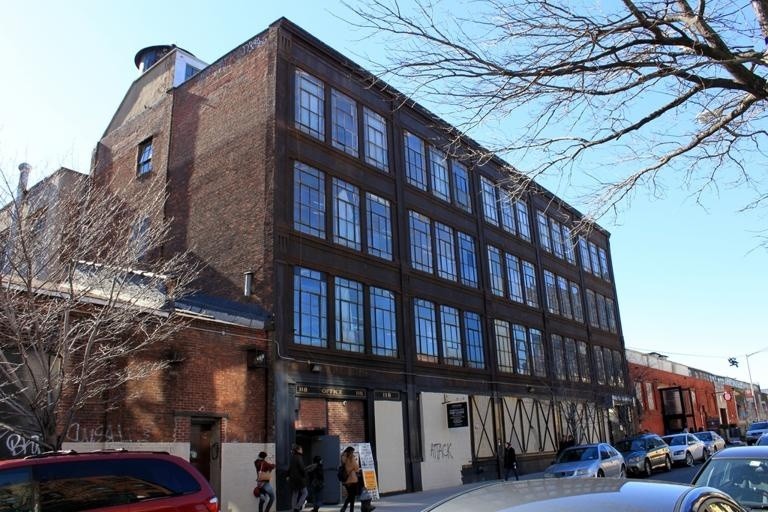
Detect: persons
[337,445,364,511]
[567,434,576,448]
[305,454,326,511]
[503,442,521,481]
[285,443,310,511]
[252,450,279,511]
[556,434,566,457]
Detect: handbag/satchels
[258,471,272,481]
[254,487,263,497]
[312,479,320,493]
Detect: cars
[662,426,709,467]
[420,476,748,511]
[693,430,726,455]
[613,432,673,477]
[688,446,768,512]
[755,432,768,445]
[544,443,627,478]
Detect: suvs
[746,421,767,444]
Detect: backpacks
[338,456,353,482]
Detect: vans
[0,446,220,510]
